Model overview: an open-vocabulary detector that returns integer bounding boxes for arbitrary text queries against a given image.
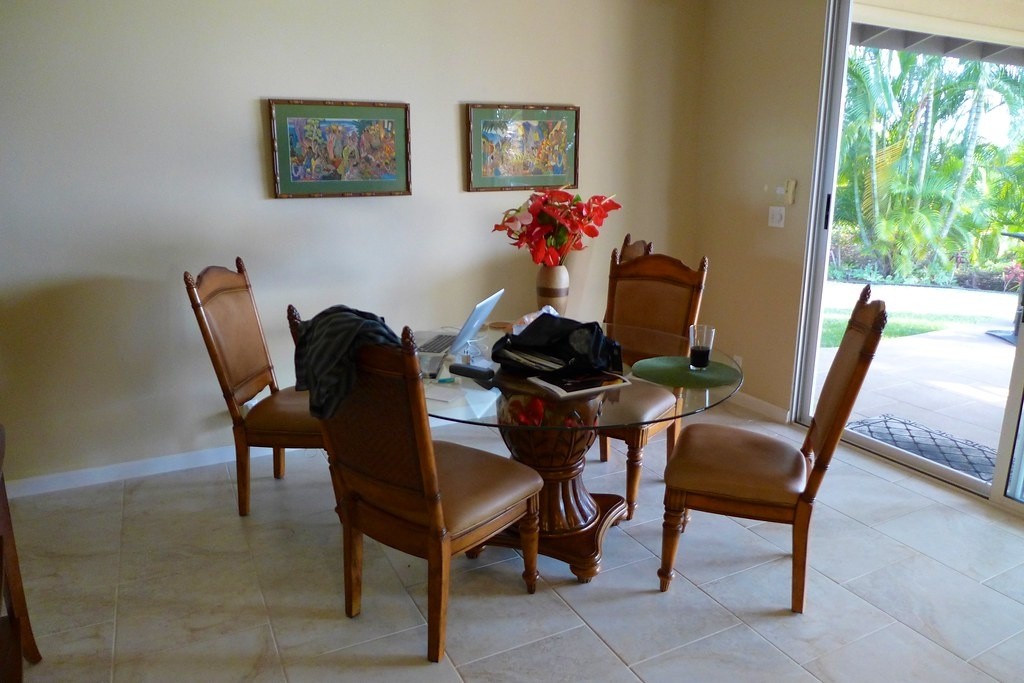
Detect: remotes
[449,364,495,380]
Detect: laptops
[414,288,505,357]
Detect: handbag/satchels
[491,312,623,378]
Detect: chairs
[597,249,709,518]
[181,257,326,516]
[283,303,544,663]
[603,234,653,325]
[657,283,887,614]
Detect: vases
[535,263,570,318]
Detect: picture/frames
[268,98,413,199]
[465,103,581,193]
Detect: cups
[689,325,716,370]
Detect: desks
[399,328,745,584]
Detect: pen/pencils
[429,378,455,384]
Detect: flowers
[490,186,624,267]
